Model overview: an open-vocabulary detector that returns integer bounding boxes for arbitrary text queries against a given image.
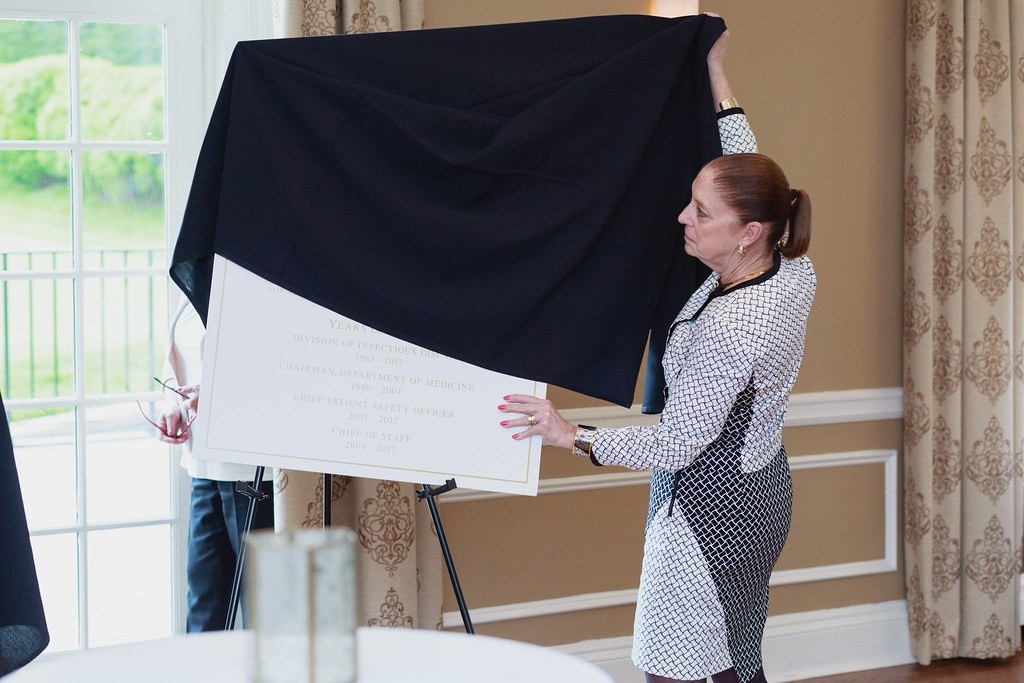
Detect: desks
[0,627,617,683]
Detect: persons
[499,11,817,683]
[159,294,274,634]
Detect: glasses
[136,377,196,438]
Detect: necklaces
[721,268,767,290]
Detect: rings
[529,415,535,423]
